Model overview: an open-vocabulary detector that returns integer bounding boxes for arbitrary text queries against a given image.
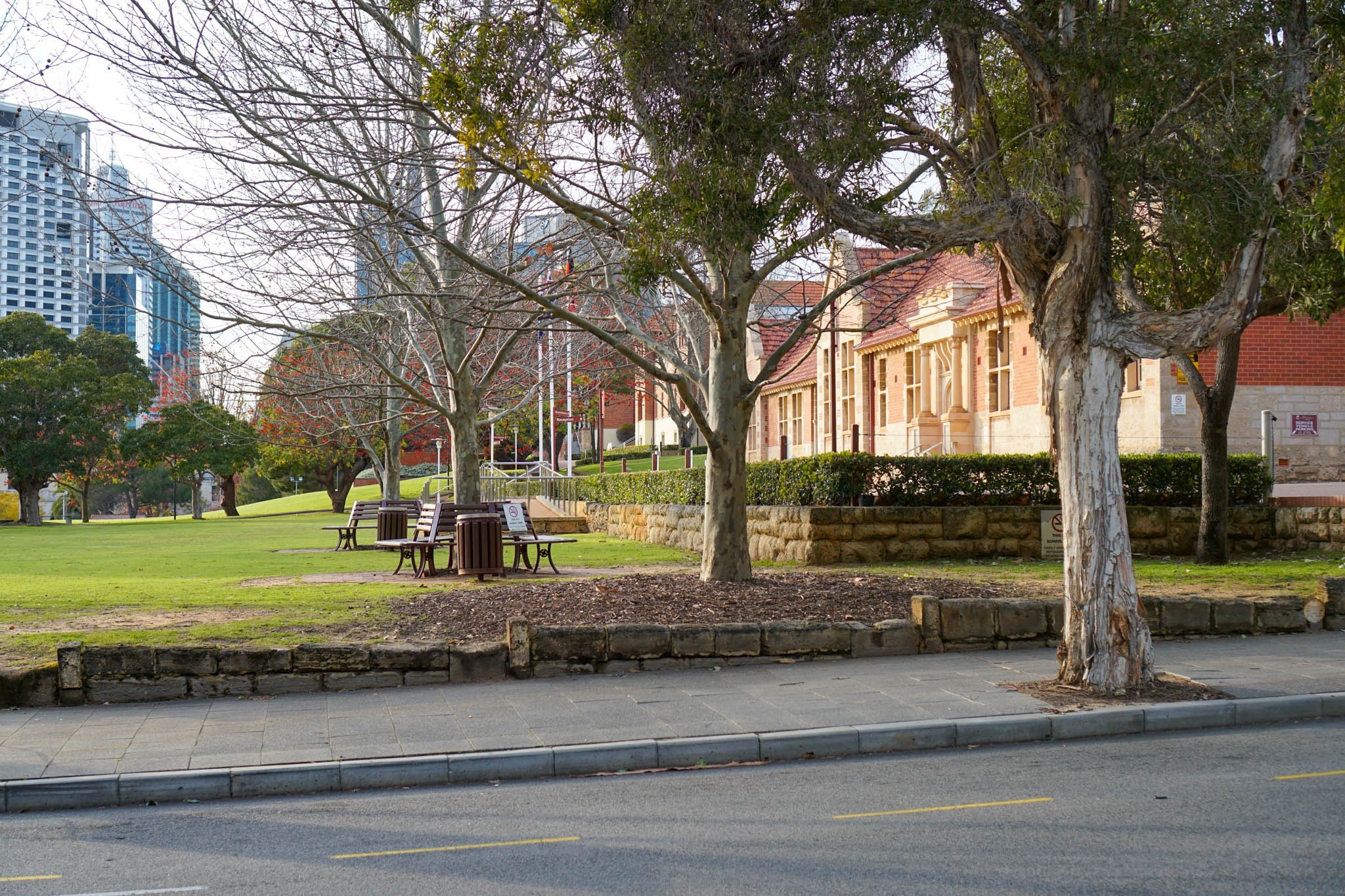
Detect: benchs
[322,500,578,577]
[41,515,72,525]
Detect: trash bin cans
[456,512,505,575]
[377,506,408,550]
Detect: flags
[548,270,562,282]
[566,247,578,312]
[537,285,557,340]
